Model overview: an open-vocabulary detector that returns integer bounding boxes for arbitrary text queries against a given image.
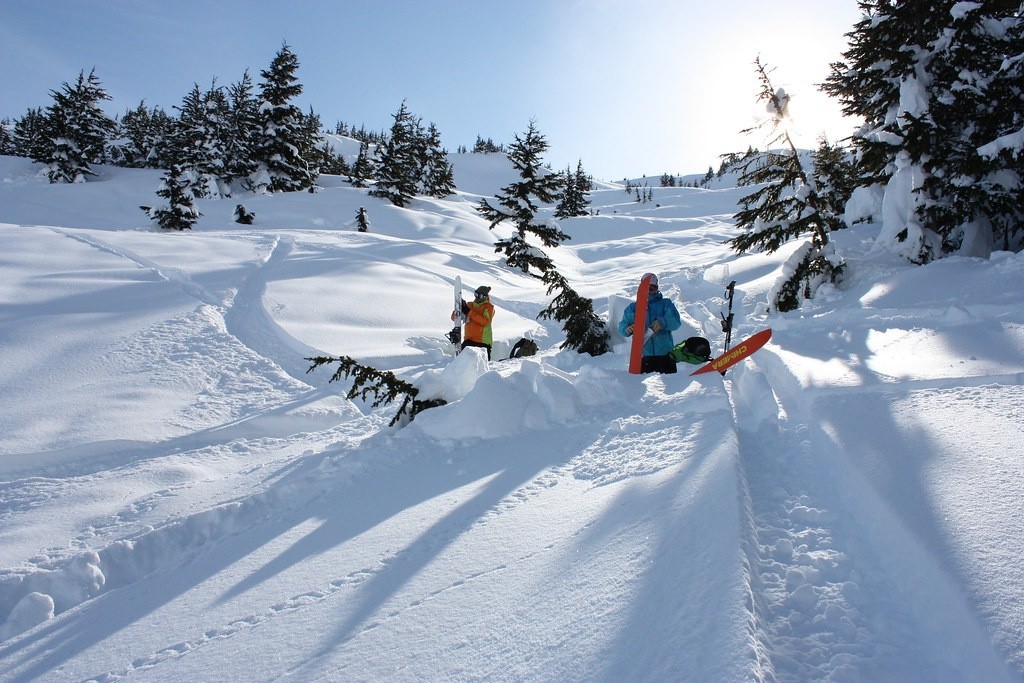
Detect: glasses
[650,286,658,290]
[474,292,487,300]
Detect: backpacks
[665,337,716,365]
[510,338,537,359]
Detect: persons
[450,286,495,361]
[618,273,681,374]
[595,209,600,215]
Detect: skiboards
[628,272,774,381]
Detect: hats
[475,286,491,295]
[641,273,658,287]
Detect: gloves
[626,324,634,334]
[462,299,470,315]
[650,319,665,332]
[451,312,456,321]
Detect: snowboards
[447,272,465,359]
